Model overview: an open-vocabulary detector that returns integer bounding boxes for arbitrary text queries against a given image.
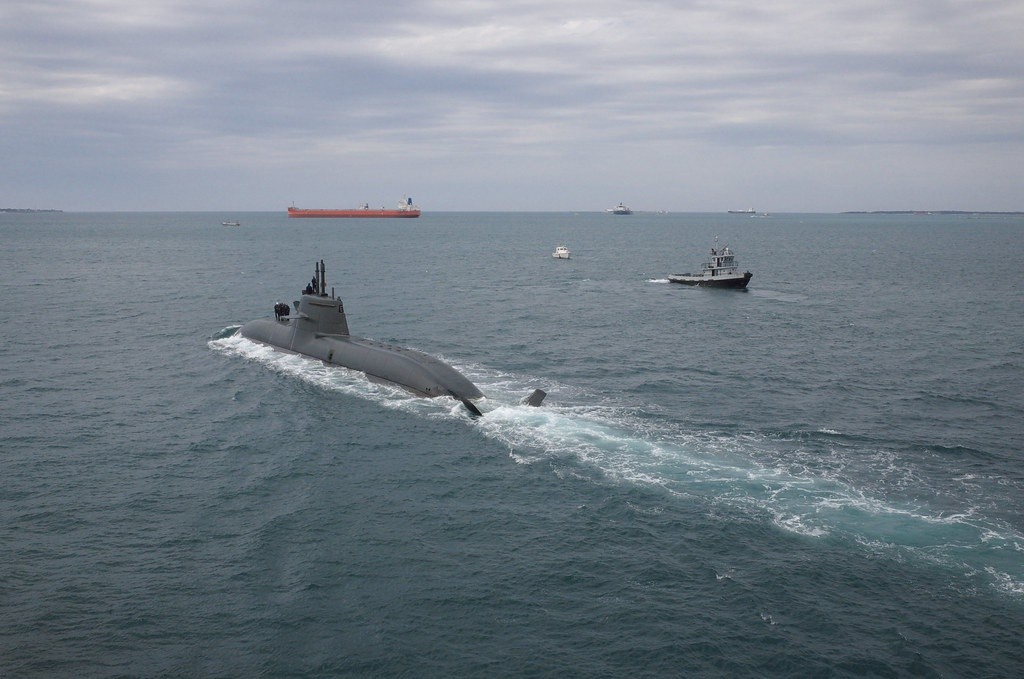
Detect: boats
[552,244,571,259]
[728,208,755,214]
[667,234,754,291]
[606,202,633,215]
[221,220,240,226]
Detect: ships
[242,256,548,417]
[287,198,421,219]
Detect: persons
[273,302,290,321]
[306,276,319,294]
